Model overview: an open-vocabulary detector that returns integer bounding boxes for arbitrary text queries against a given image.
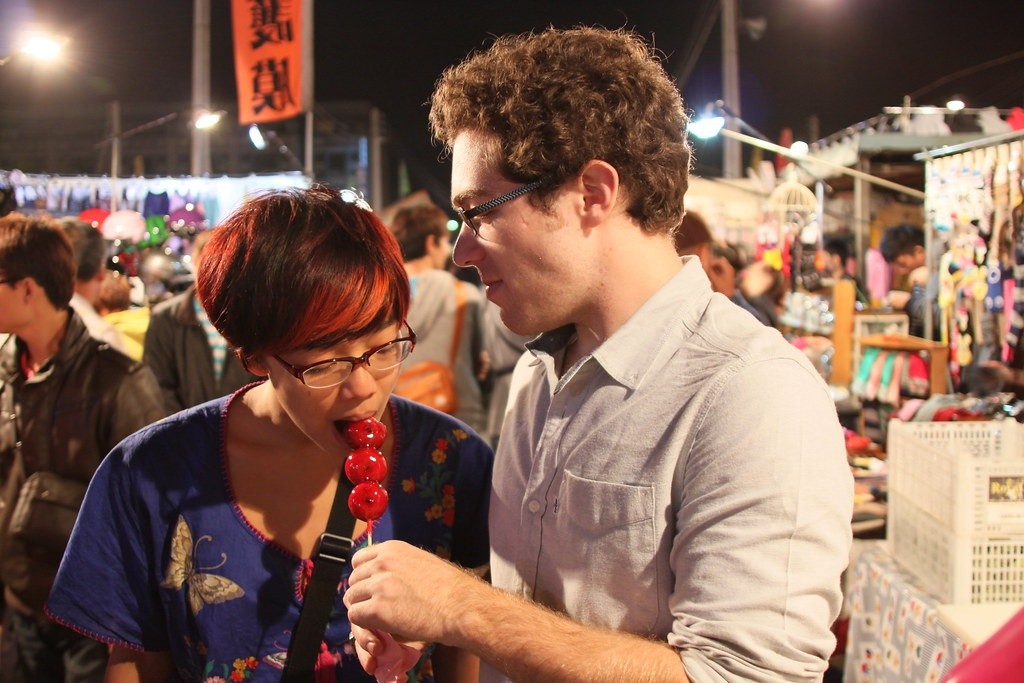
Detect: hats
[674,212,713,250]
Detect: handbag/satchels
[10,471,86,545]
[395,361,457,413]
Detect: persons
[45,189,494,683]
[343,27,856,683]
[55,216,128,355]
[0,212,167,683]
[980,322,1024,396]
[882,226,925,312]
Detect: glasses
[456,181,540,235]
[269,319,416,389]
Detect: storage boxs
[886,417,1024,605]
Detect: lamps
[121,107,228,140]
[689,100,834,196]
[248,124,302,172]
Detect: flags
[233,0,304,126]
